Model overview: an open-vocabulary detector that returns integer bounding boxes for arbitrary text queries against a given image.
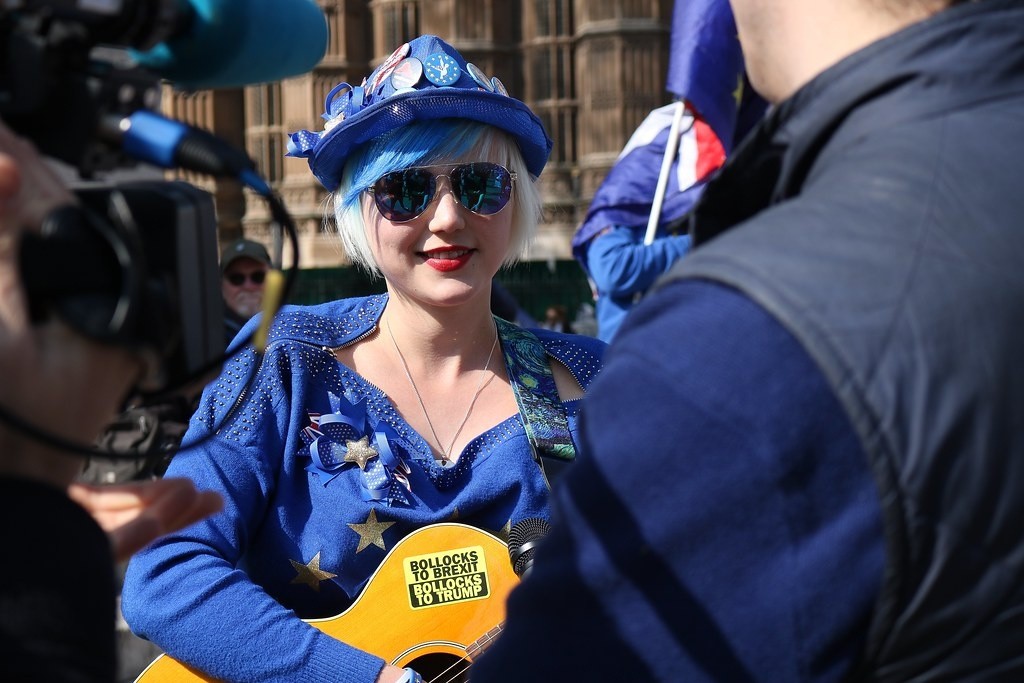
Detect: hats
[219,240,271,273]
[285,34,554,193]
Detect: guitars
[131,523,522,683]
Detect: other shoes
[401,205,409,210]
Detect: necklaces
[384,309,498,468]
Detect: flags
[665,0,747,193]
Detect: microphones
[507,518,552,578]
[51,0,329,93]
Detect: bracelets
[397,667,422,683]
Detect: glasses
[364,160,518,224]
[223,270,265,287]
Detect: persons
[222,241,272,354]
[469,1,1023,683]
[538,306,575,335]
[0,1,330,683]
[571,87,768,344]
[122,34,610,683]
